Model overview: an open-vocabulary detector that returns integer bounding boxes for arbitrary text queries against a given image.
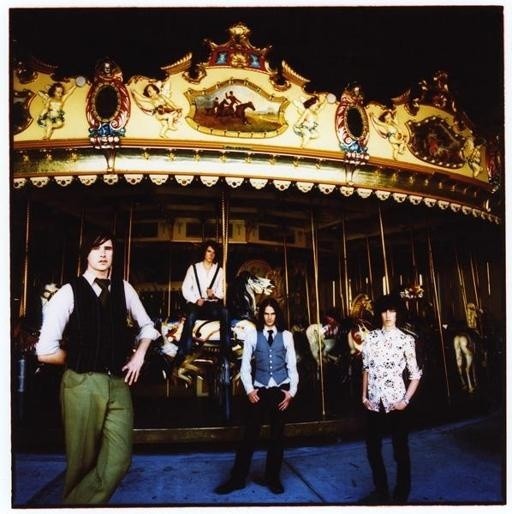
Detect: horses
[222,102,256,125]
[204,99,228,120]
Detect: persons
[172,238,237,371]
[356,293,424,503]
[36,230,162,503]
[213,296,301,495]
